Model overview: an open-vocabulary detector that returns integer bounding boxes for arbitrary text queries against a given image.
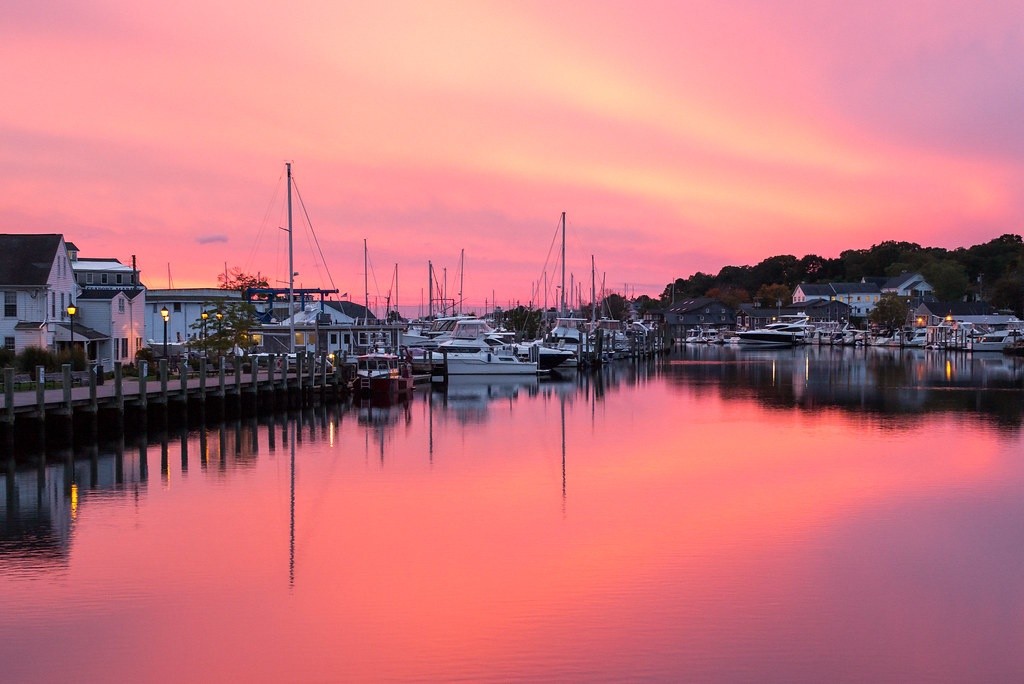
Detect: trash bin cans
[92,365,105,386]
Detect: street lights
[162,315,170,358]
[161,305,168,357]
[67,302,76,349]
[215,312,223,356]
[202,310,209,356]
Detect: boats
[245,161,1024,373]
